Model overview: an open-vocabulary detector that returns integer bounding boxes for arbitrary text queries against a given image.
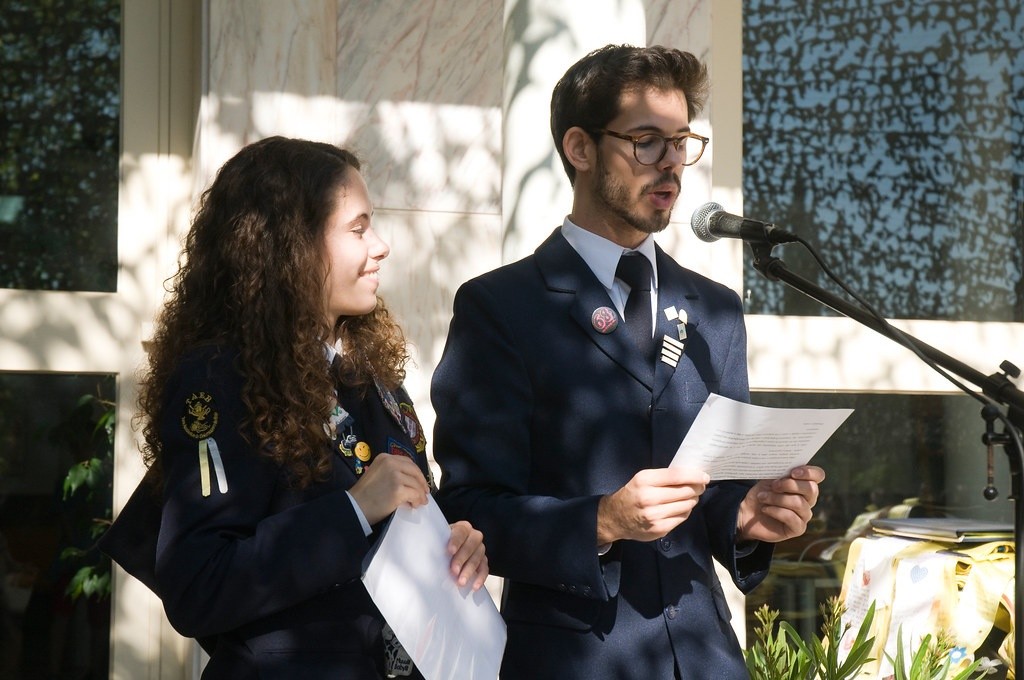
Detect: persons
[429,48,829,679]
[103,136,489,679]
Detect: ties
[615,255,658,372]
[329,354,355,416]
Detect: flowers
[746,597,1000,680]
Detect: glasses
[584,125,708,166]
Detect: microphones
[690,202,798,245]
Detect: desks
[816,534,1018,680]
